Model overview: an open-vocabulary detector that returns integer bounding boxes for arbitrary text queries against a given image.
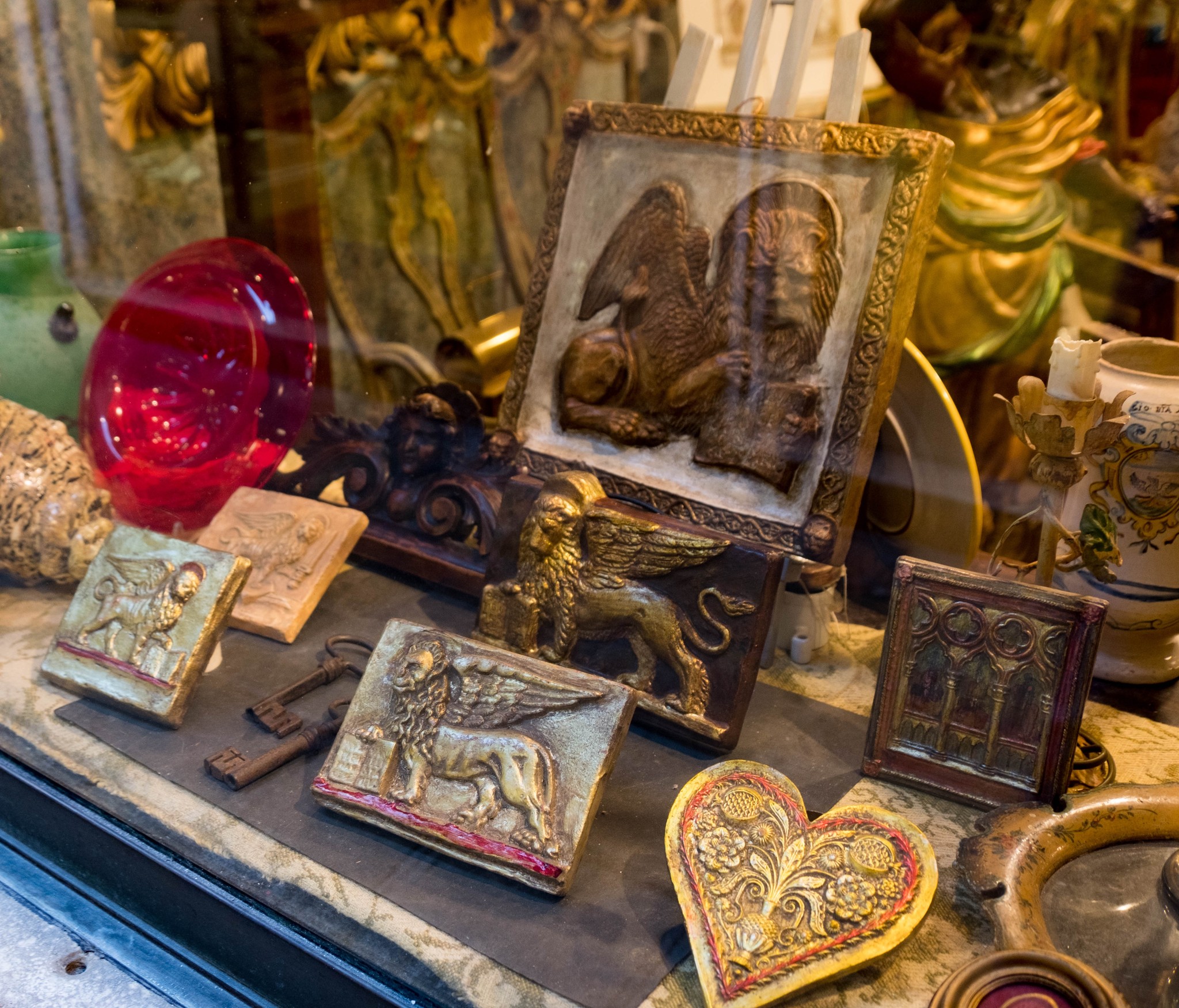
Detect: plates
[79,239,319,531]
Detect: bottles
[1053,337,1179,684]
[1,229,105,445]
